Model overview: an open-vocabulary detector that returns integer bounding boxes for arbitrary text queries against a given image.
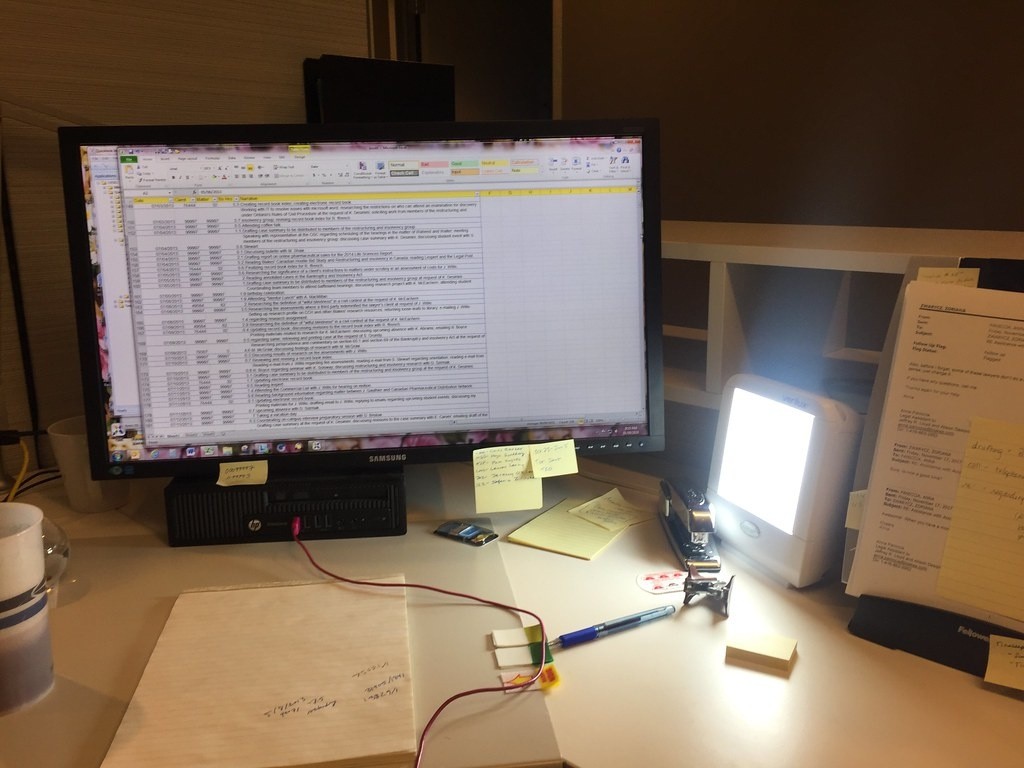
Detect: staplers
[655,474,722,572]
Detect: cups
[47,415,128,513]
[0,502,54,717]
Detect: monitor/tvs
[706,372,863,586]
[50,115,664,482]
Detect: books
[100,575,418,768]
[844,279,1024,638]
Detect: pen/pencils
[549,605,677,650]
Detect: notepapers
[490,624,547,647]
[494,643,553,669]
[724,628,799,672]
[499,662,561,694]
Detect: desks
[0,433,1024,768]
